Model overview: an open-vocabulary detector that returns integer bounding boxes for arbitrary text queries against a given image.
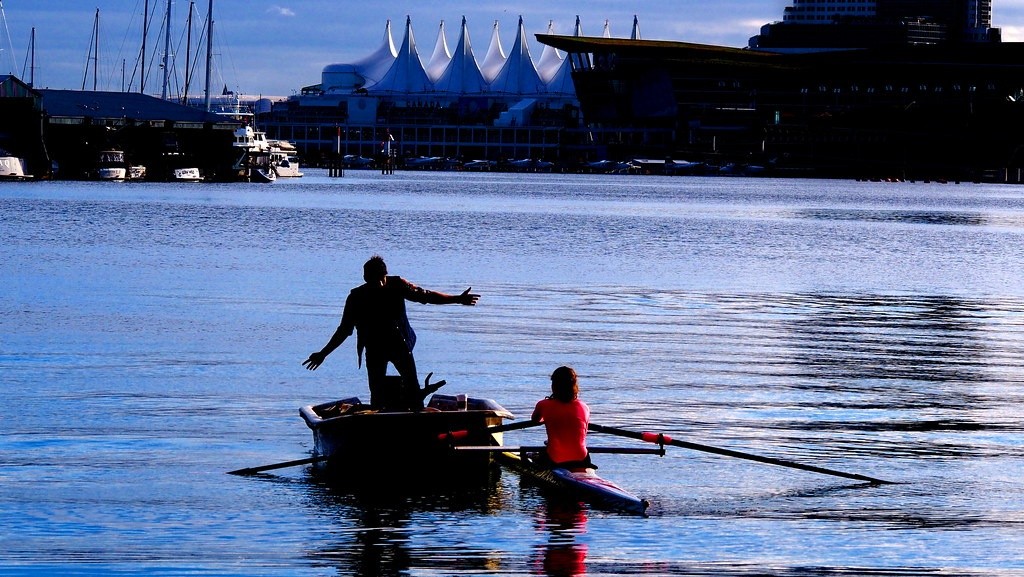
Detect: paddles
[227,417,546,475]
[587,422,900,485]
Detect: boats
[0,156,34,181]
[267,140,304,177]
[502,451,650,513]
[299,372,515,482]
[233,126,276,182]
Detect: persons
[532,366,593,469]
[302,256,482,412]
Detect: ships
[534,0,1024,180]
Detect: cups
[457,394,467,411]
